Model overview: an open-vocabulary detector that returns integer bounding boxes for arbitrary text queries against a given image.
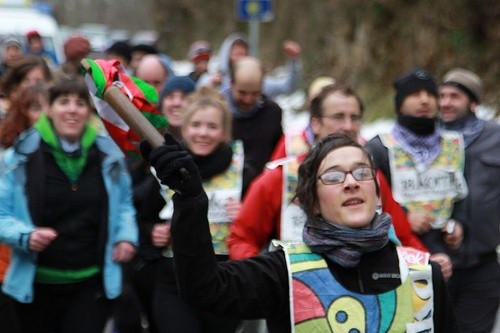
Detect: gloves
[139,132,203,197]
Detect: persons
[1,31,500,333]
[139,131,461,333]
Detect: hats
[393,68,439,111]
[445,69,483,106]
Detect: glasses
[317,166,379,186]
